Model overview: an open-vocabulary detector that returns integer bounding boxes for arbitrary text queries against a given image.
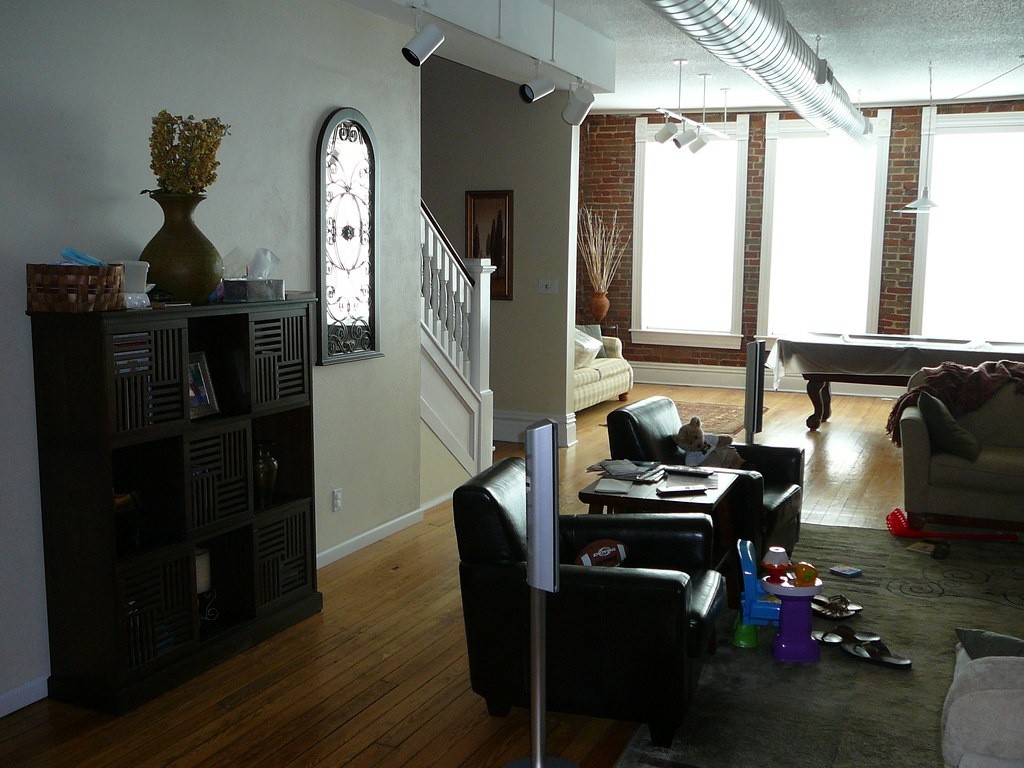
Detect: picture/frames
[465,190,514,300]
[187,351,220,420]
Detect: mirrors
[317,107,385,364]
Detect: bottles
[252,448,278,504]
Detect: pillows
[576,323,608,359]
[916,390,982,463]
[954,628,1024,660]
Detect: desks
[577,467,739,581]
[582,321,619,338]
[776,330,1024,431]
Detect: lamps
[401,0,595,128]
[655,56,730,151]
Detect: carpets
[608,521,1024,768]
[596,399,773,435]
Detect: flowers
[148,109,230,193]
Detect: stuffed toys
[670,417,746,469]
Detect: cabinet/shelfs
[22,294,322,706]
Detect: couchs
[899,367,1024,529]
[572,334,633,413]
[608,393,807,584]
[455,455,723,749]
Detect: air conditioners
[892,63,954,216]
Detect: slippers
[811,592,864,619]
[813,623,912,670]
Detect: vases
[252,438,277,501]
[591,290,610,323]
[138,189,224,305]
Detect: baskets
[26,263,125,313]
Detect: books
[584,458,666,493]
